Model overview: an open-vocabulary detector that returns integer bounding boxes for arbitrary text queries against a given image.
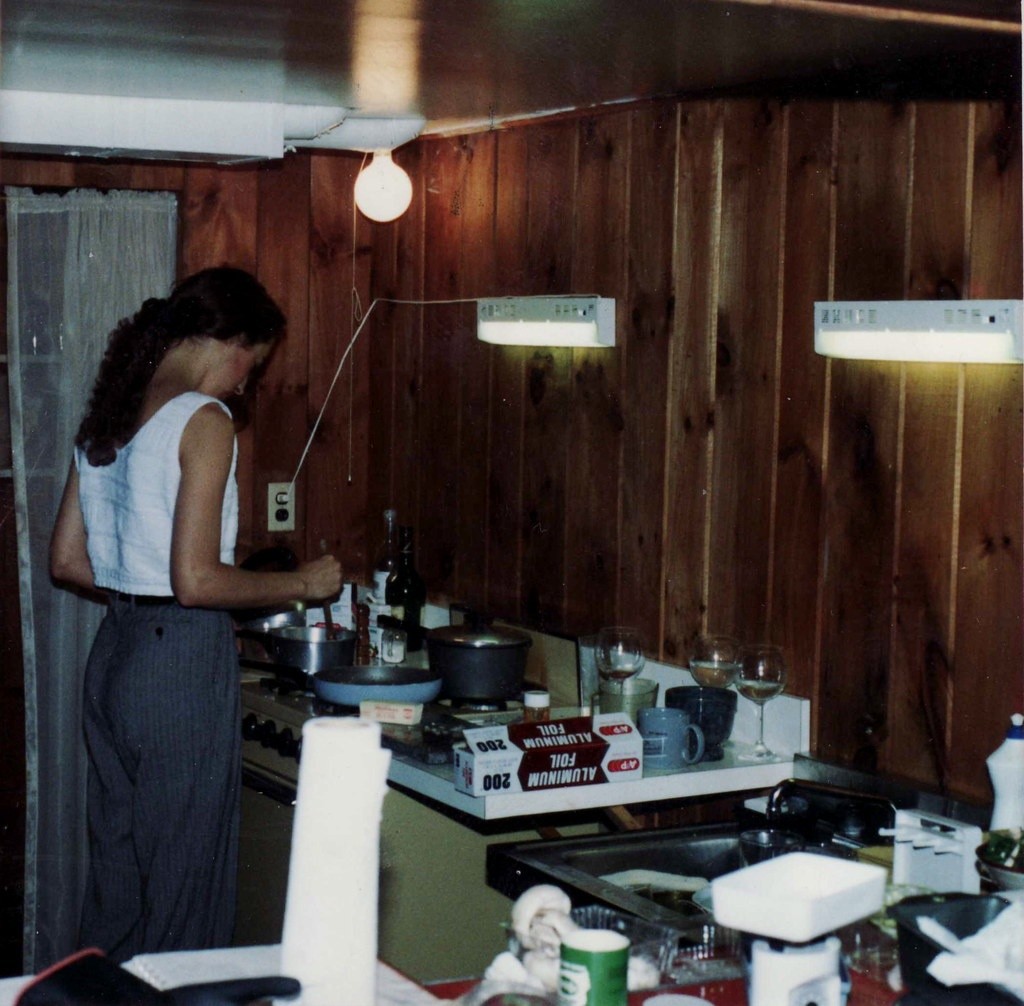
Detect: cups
[636,707,704,770]
[557,927,630,1006]
[590,677,659,725]
[739,829,804,869]
[523,690,550,724]
[665,686,737,762]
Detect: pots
[235,625,357,676]
[376,614,533,699]
[259,661,455,706]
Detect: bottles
[986,713,1024,840]
[372,510,399,601]
[385,523,426,651]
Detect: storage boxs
[712,850,887,944]
[452,712,644,794]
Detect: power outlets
[266,483,295,533]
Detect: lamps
[478,297,616,347]
[352,149,415,224]
[814,298,1024,364]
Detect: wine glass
[689,633,741,688]
[594,627,645,694]
[734,642,789,762]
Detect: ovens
[230,760,297,948]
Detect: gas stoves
[237,597,581,783]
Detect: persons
[52,263,346,957]
